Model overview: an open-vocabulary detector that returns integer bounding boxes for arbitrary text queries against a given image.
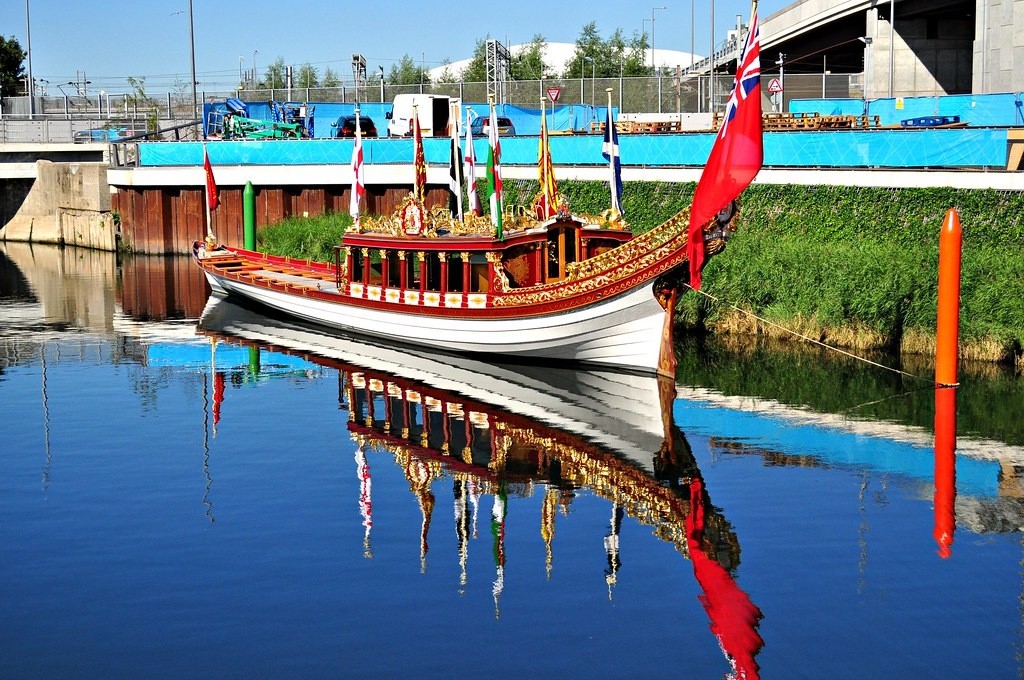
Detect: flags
[212,371,226,438]
[464,113,484,216]
[538,101,559,222]
[348,128,367,232]
[413,114,427,207]
[485,106,504,228]
[685,7,765,290]
[448,119,464,223]
[602,107,625,217]
[356,445,622,614]
[202,152,218,210]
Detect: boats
[195,292,767,680]
[191,1,764,378]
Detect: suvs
[74,118,163,144]
[330,114,378,139]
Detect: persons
[223,116,230,139]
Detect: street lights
[240,56,244,87]
[584,55,595,107]
[652,7,667,67]
[642,18,655,69]
[254,50,257,84]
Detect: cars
[465,115,516,137]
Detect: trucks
[386,94,462,138]
